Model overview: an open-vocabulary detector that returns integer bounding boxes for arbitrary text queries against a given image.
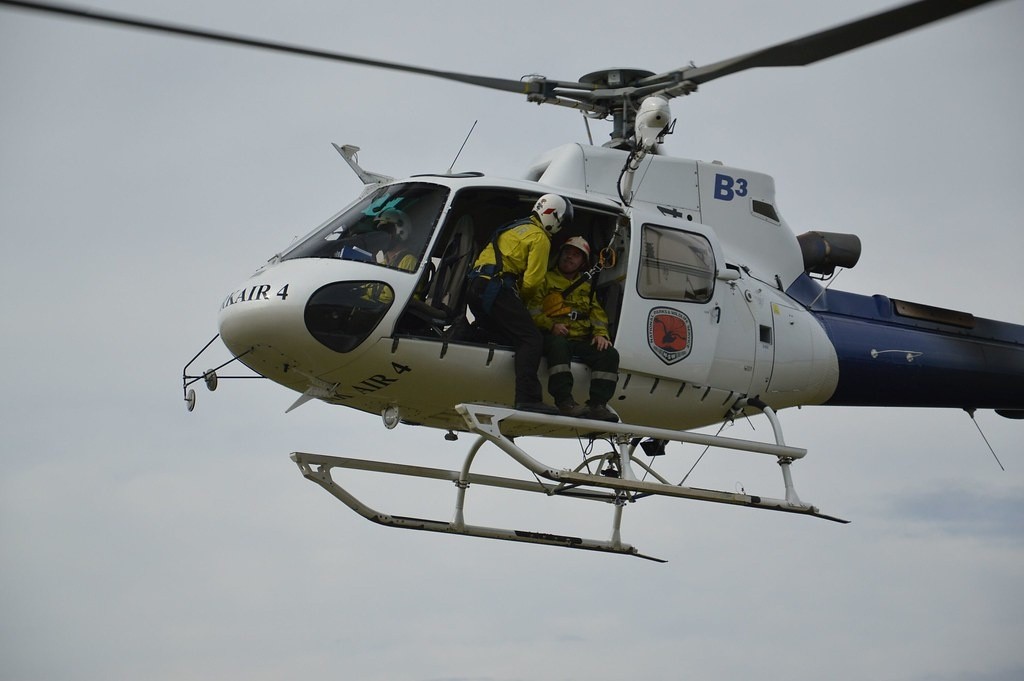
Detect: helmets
[560,236,590,262]
[377,209,412,240]
[532,194,574,233]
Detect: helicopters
[1,0,1024,565]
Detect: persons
[360,210,422,308]
[467,192,573,415]
[528,236,619,422]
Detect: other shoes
[555,400,619,423]
[515,400,559,413]
